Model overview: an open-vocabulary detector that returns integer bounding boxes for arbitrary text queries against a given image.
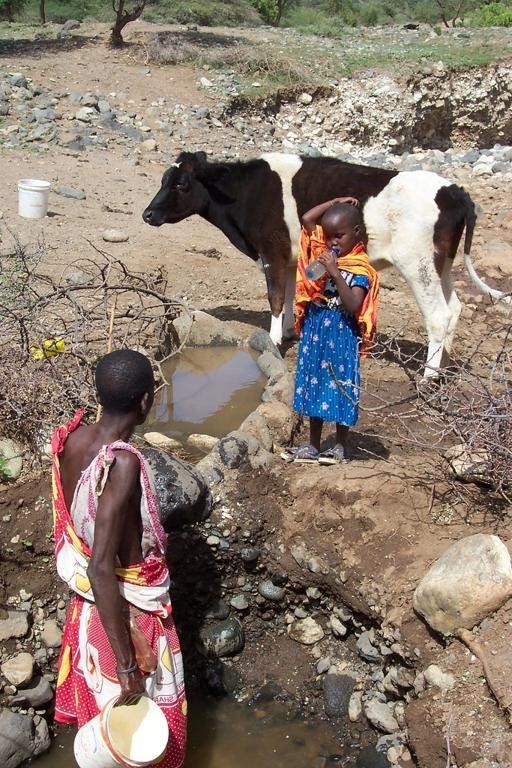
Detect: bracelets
[115,663,137,675]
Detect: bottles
[305,244,340,280]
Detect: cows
[143,149,512,392]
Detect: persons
[282,198,381,467]
[48,351,187,768]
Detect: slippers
[294,444,345,464]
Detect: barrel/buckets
[17,179,51,218]
[73,694,170,768]
